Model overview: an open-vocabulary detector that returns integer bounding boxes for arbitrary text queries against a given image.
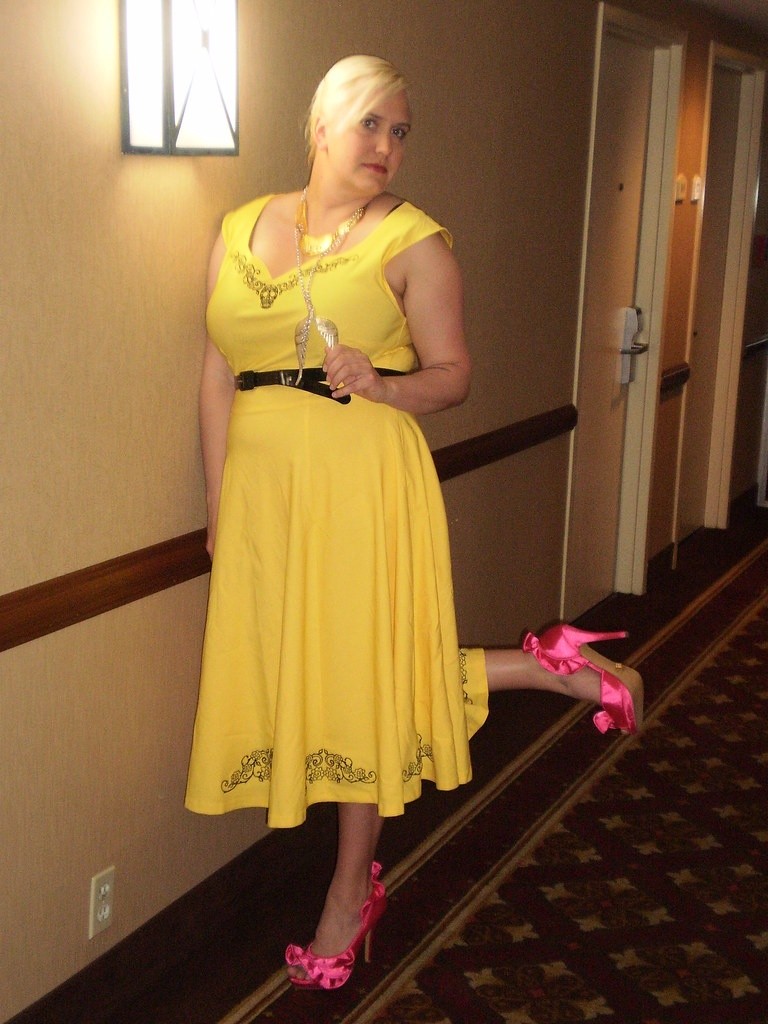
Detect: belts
[234,361,404,405]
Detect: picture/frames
[118,0,240,157]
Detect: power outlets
[89,864,116,940]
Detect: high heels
[523,623,644,736]
[285,855,387,990]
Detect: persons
[183,55,644,990]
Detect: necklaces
[293,185,365,386]
